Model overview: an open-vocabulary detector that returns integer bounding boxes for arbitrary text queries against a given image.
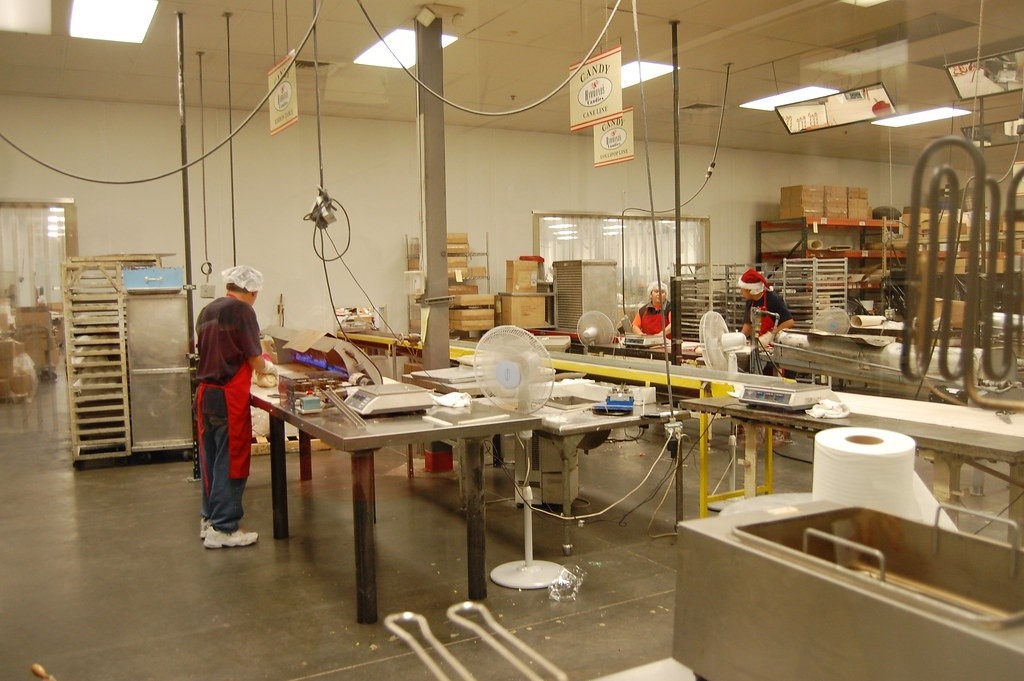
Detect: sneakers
[201,518,258,548]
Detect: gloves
[256,360,278,379]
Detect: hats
[738,268,774,291]
[222,265,263,292]
[648,281,668,299]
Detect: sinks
[731,504,1024,632]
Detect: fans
[700,311,747,512]
[815,306,850,389]
[473,325,568,590]
[577,310,615,350]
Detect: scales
[621,334,667,348]
[343,385,434,415]
[737,379,841,412]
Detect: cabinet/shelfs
[756,219,1024,325]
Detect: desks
[250,370,543,624]
[476,399,692,531]
[679,390,1024,550]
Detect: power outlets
[664,422,682,441]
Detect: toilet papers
[811,427,959,534]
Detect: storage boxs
[779,184,1024,328]
[506,260,537,293]
[446,233,494,330]
[0,303,63,401]
[495,296,546,329]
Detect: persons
[738,270,794,347]
[196,265,280,548]
[631,282,673,340]
